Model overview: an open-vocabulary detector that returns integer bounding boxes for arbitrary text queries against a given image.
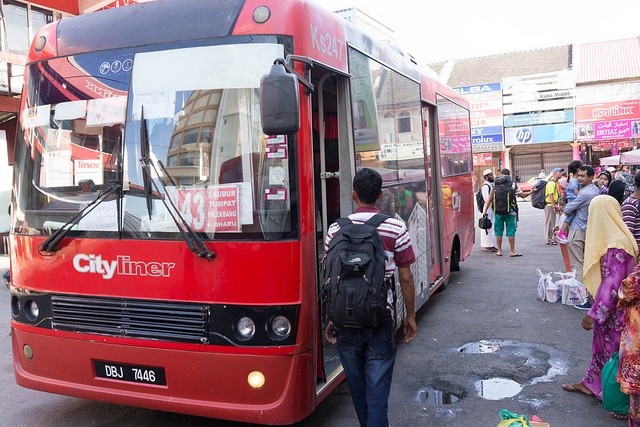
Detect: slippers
[509,252,524,257]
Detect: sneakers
[573,296,593,311]
[555,229,569,245]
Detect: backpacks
[492,176,515,215]
[322,213,391,329]
[532,179,556,209]
[475,183,491,213]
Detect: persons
[544,167,564,245]
[607,179,626,205]
[620,171,640,256]
[556,160,582,244]
[480,169,498,251]
[482,169,537,257]
[516,170,521,183]
[564,166,599,282]
[393,184,418,226]
[322,168,420,427]
[562,194,639,401]
[617,262,640,426]
[606,166,628,184]
[539,167,545,177]
[594,170,612,195]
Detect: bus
[4,0,476,425]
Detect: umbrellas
[598,147,640,168]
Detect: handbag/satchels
[478,213,491,235]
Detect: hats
[482,168,494,176]
[551,167,565,175]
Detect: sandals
[561,382,597,398]
